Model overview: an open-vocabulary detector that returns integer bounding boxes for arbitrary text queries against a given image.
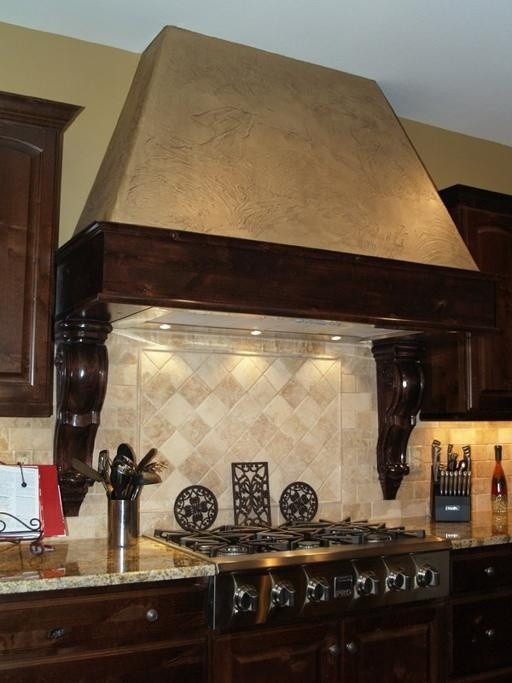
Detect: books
[1,462,71,541]
[0,463,48,540]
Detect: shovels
[71,456,108,497]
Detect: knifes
[431,439,472,496]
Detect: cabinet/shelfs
[212,601,442,681]
[445,545,510,681]
[411,184,511,421]
[0,88,86,415]
[2,581,210,681]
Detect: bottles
[490,446,508,514]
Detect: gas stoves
[143,514,453,633]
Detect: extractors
[59,24,503,345]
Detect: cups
[107,498,138,547]
